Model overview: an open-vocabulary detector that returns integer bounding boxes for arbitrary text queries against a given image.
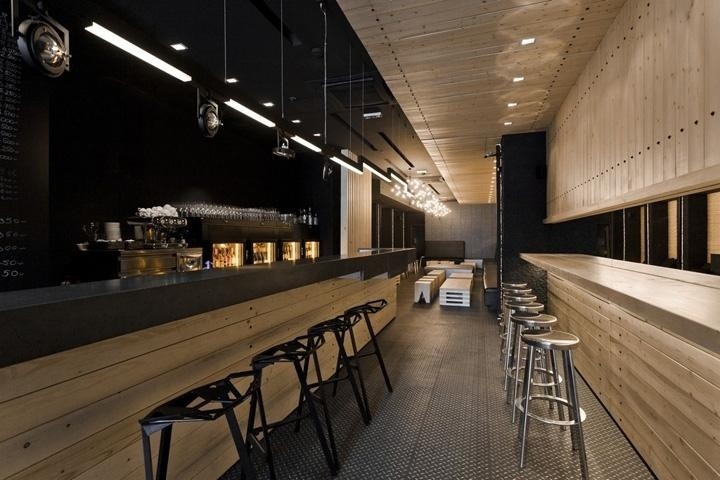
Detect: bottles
[212,242,321,268]
[299,207,319,226]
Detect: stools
[246,333,336,476]
[332,299,394,414]
[414,270,445,304]
[137,369,273,478]
[499,280,590,480]
[294,312,369,434]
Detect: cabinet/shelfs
[83,217,318,274]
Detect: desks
[438,272,474,308]
[518,252,720,480]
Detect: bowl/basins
[105,222,122,241]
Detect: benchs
[484,258,500,312]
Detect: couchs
[425,258,484,278]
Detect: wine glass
[173,202,281,225]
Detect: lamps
[82,20,414,202]
